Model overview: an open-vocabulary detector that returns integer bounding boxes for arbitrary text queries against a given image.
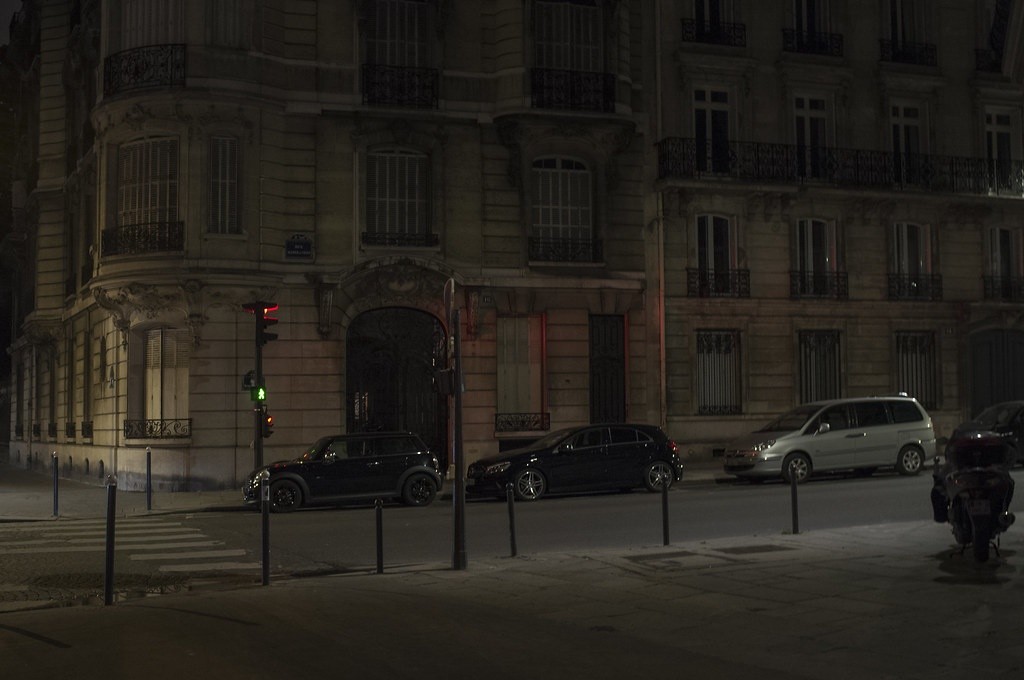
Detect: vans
[723,395,937,487]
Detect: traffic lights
[242,303,256,313]
[262,414,274,438]
[256,301,278,345]
[250,386,266,400]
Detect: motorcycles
[931,409,1016,565]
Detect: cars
[945,400,1024,463]
[241,432,444,512]
[467,422,683,501]
